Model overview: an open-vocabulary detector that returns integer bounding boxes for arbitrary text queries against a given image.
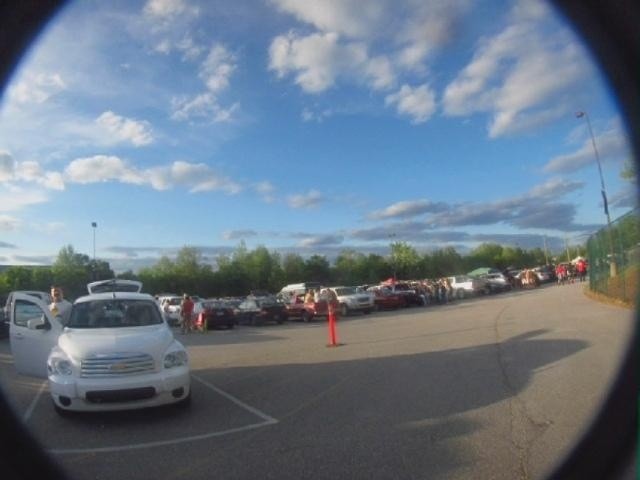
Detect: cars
[0,279,197,417]
[196,262,577,333]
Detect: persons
[39,283,73,330]
[383,275,453,306]
[153,292,208,336]
[499,252,629,289]
[275,287,337,306]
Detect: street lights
[91,222,97,259]
[575,111,615,263]
